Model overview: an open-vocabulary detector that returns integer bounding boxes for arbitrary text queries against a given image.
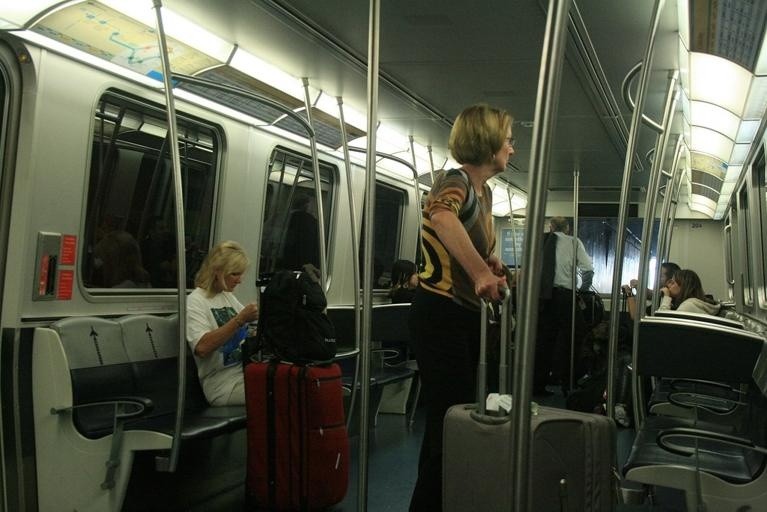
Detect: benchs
[30,302,415,512]
[623,308,766,512]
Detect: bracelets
[626,292,633,296]
[234,315,244,328]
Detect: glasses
[505,138,519,150]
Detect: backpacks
[254,264,341,367]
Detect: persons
[621,262,682,321]
[509,271,521,314]
[97,230,209,288]
[407,102,516,511]
[533,215,594,398]
[183,242,259,405]
[601,269,722,427]
[383,260,420,365]
[288,195,323,271]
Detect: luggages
[243,357,352,511]
[438,275,622,511]
